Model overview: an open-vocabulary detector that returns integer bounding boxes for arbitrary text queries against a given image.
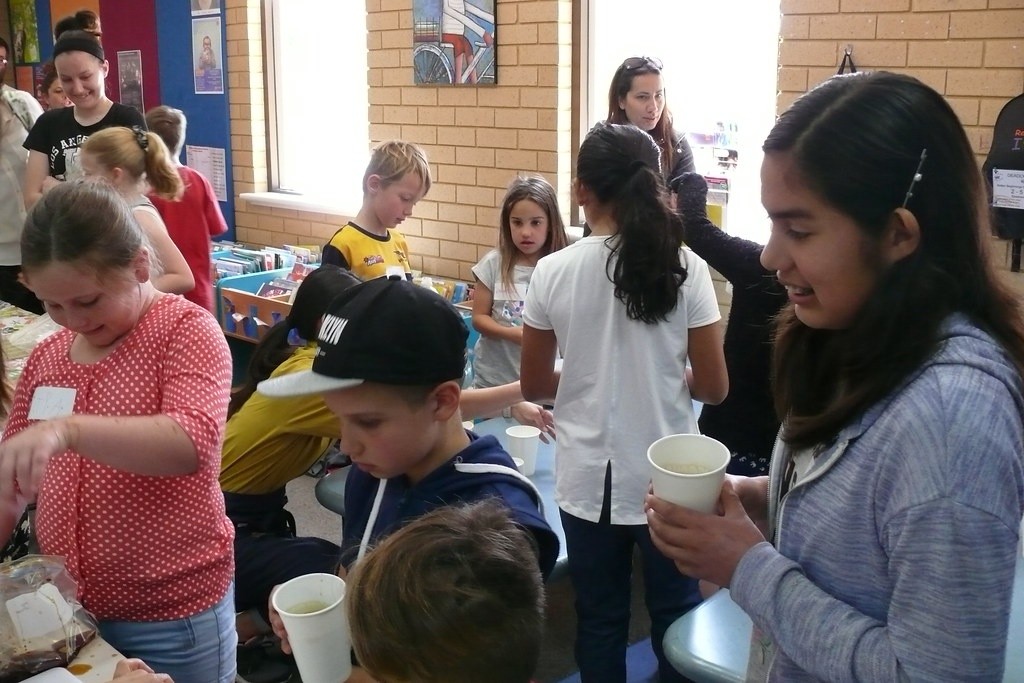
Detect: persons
[644,71,1024,683]
[0,11,788,683]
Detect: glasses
[620,56,663,78]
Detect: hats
[256,273,470,396]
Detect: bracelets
[503,406,512,421]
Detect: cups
[505,426,541,477]
[462,420,475,432]
[272,573,351,683]
[648,433,731,515]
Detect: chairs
[662,513,1023,683]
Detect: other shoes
[236,639,294,683]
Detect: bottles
[420,276,440,295]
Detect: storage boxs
[218,263,323,346]
[211,237,248,319]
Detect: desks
[311,412,570,586]
